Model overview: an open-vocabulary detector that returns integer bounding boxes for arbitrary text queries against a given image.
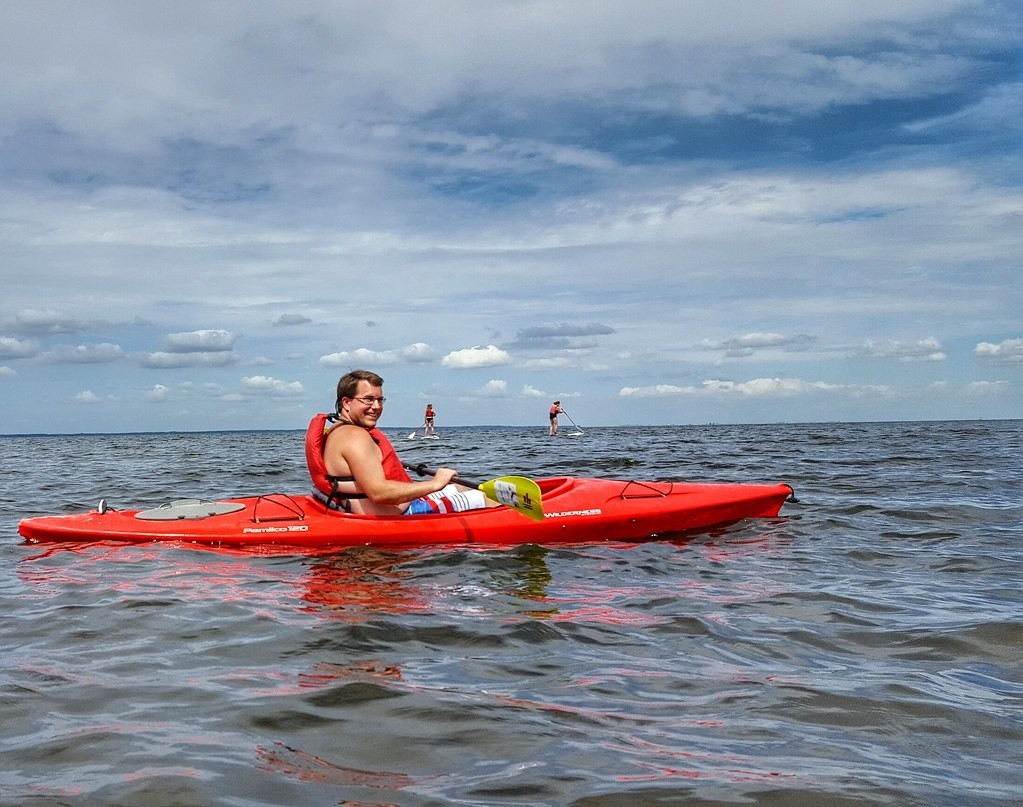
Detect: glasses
[346,396,387,405]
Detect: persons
[549,401,565,435]
[425,403,436,438]
[322,369,504,515]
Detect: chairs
[310,486,347,514]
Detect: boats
[13,475,800,549]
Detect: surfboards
[564,432,583,436]
[420,436,440,440]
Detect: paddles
[322,427,544,522]
[561,408,584,432]
[407,422,426,440]
